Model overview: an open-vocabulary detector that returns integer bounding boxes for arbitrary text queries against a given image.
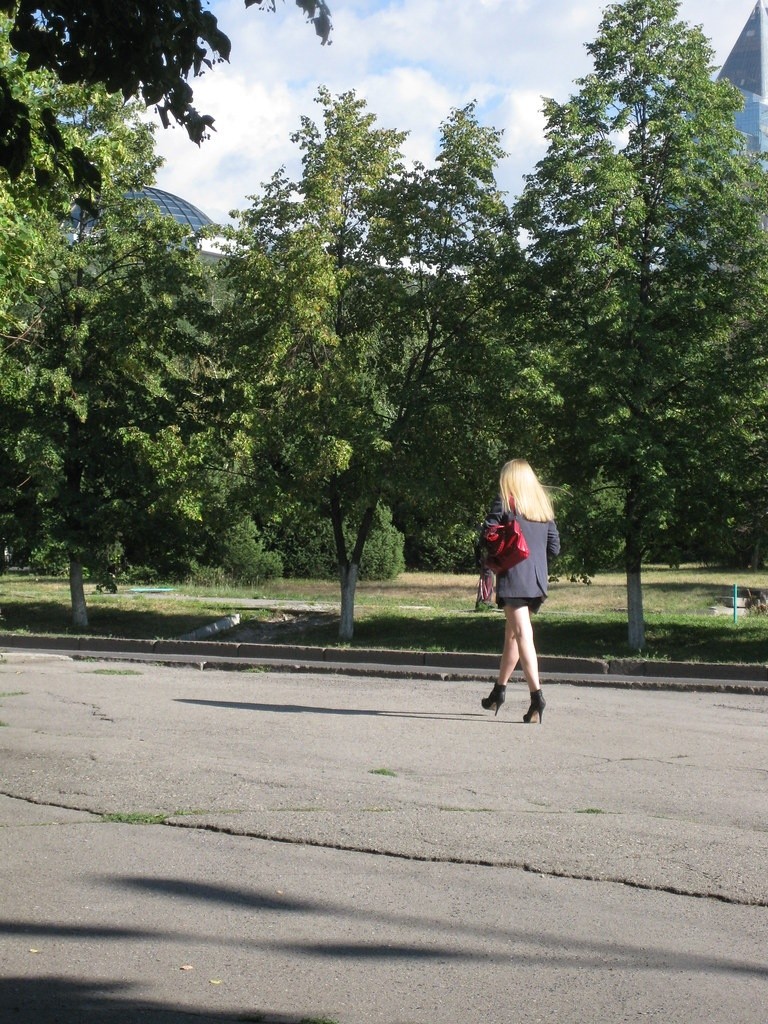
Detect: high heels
[482,683,506,716]
[523,690,546,724]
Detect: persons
[478,459,561,725]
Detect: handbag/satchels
[484,497,529,573]
